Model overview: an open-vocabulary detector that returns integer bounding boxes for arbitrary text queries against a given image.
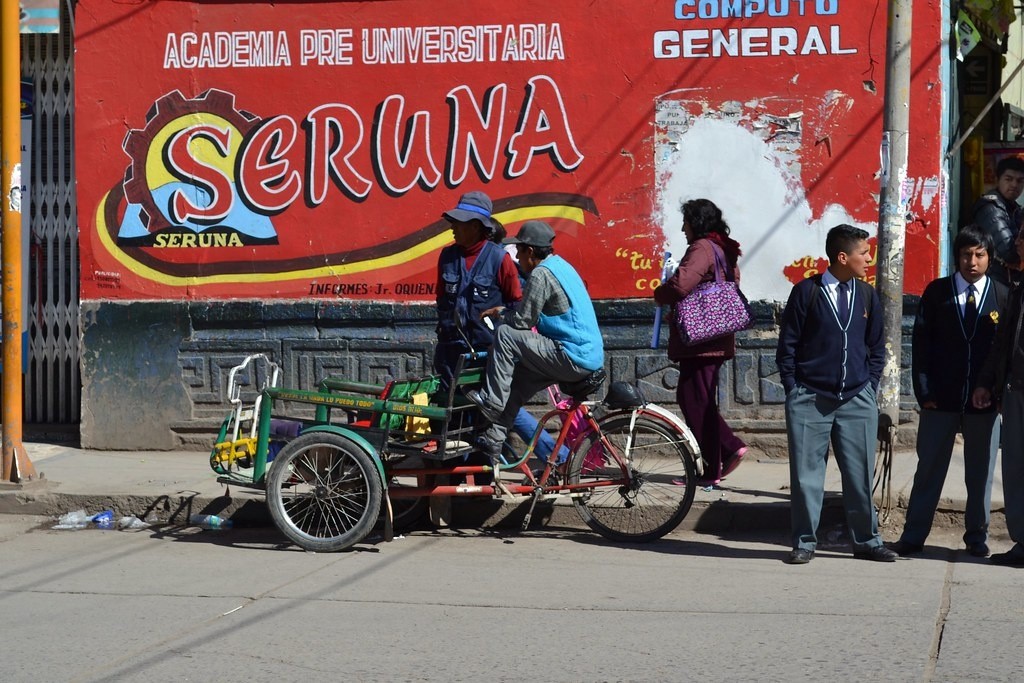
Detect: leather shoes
[789,547,815,563]
[854,544,899,562]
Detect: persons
[434,190,581,497]
[880,224,1024,570]
[465,219,606,465]
[956,155,1024,287]
[652,199,749,488]
[774,224,900,565]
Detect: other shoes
[989,550,1024,566]
[966,543,991,556]
[720,447,747,478]
[461,383,500,422]
[462,431,503,459]
[887,540,926,556]
[672,474,719,485]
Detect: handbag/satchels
[670,238,756,347]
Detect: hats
[441,190,497,232]
[502,221,556,247]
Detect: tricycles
[211,334,705,553]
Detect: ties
[1011,297,1024,362]
[964,285,976,341]
[838,282,849,330]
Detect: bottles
[189,515,231,526]
